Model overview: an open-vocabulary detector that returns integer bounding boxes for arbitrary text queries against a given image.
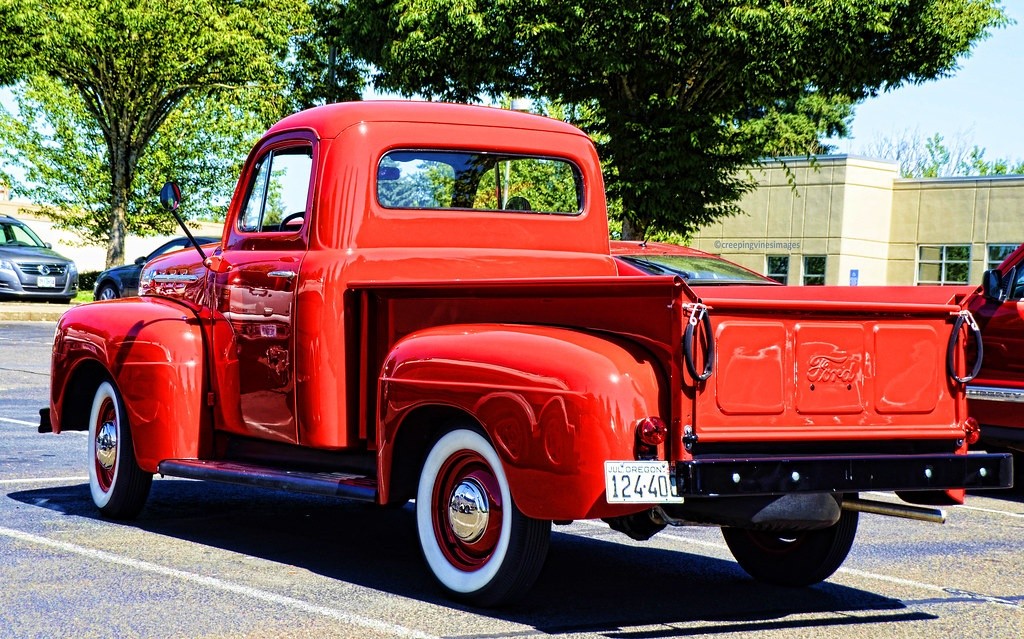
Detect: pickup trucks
[39,99,1016,613]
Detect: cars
[0,214,80,303]
[93,235,222,302]
[889,243,1024,506]
[608,238,785,285]
